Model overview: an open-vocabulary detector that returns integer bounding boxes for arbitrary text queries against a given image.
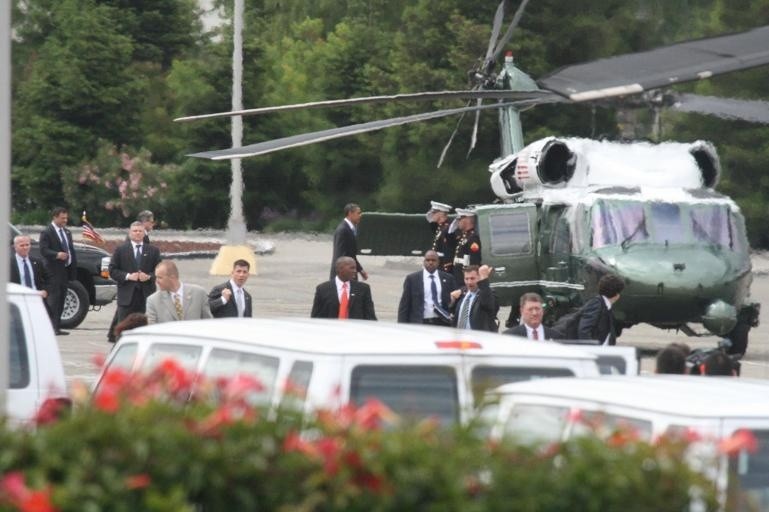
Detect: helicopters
[166,1,769,360]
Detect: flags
[81,215,105,244]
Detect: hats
[430,199,452,214]
[455,206,477,219]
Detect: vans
[5,281,69,431]
[458,371,768,511]
[82,306,646,452]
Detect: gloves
[425,211,433,222]
[448,219,460,235]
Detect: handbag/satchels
[552,314,580,340]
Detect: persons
[328,202,368,281]
[310,255,377,321]
[10,235,49,300]
[108,220,160,322]
[143,260,212,325]
[38,206,77,335]
[397,250,500,332]
[656,341,737,377]
[106,209,154,341]
[208,259,252,318]
[424,201,481,284]
[502,274,625,346]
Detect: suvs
[8,219,120,331]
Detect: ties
[236,289,244,318]
[135,245,142,267]
[607,312,616,346]
[458,294,472,328]
[429,275,442,308]
[338,283,349,319]
[533,328,539,340]
[59,228,69,266]
[174,293,186,321]
[22,257,32,288]
